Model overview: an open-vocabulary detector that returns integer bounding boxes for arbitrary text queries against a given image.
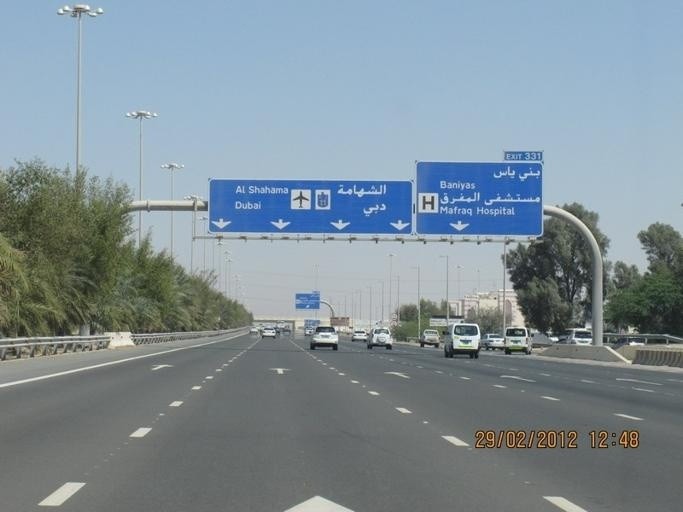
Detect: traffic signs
[414,151,543,237]
[208,179,413,234]
[295,291,320,309]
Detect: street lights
[160,164,183,264]
[182,195,244,301]
[343,254,463,343]
[125,111,158,249]
[56,5,103,176]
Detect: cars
[249,325,392,350]
[419,324,646,358]
